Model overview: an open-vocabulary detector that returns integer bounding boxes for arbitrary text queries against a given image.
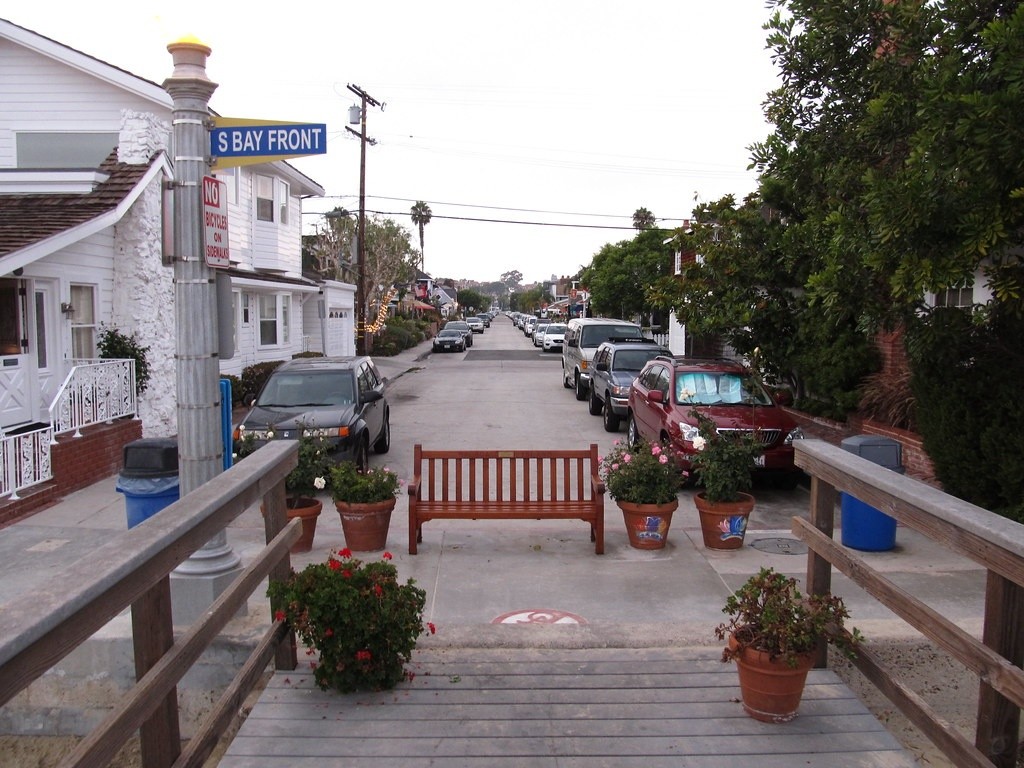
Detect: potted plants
[713,566,865,724]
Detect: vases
[616,495,678,550]
[694,492,755,551]
[335,496,396,551]
[260,498,322,555]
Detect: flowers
[329,460,405,501]
[598,433,689,508]
[666,347,781,506]
[264,548,438,693]
[232,414,335,510]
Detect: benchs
[408,444,605,556]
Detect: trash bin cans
[840,434,906,552]
[116,437,180,531]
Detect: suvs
[232,354,392,475]
[439,321,474,347]
[560,316,646,400]
[542,323,566,353]
[464,316,484,334]
[588,336,675,432]
[624,352,805,490]
[476,313,491,328]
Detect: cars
[485,309,554,347]
[432,329,467,352]
